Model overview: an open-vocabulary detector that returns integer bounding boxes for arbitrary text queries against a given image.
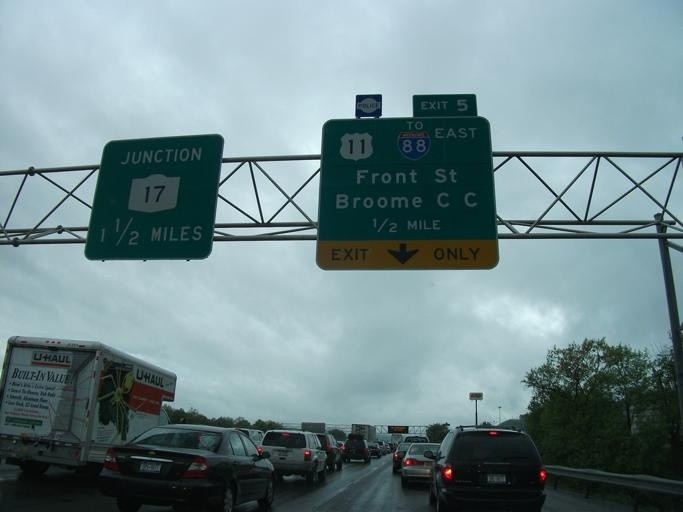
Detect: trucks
[0,335,177,489]
[350,423,377,441]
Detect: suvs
[420,422,552,512]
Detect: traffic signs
[317,92,499,270]
[84,134,224,260]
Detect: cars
[94,422,281,512]
[228,428,445,488]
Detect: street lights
[498,407,502,423]
